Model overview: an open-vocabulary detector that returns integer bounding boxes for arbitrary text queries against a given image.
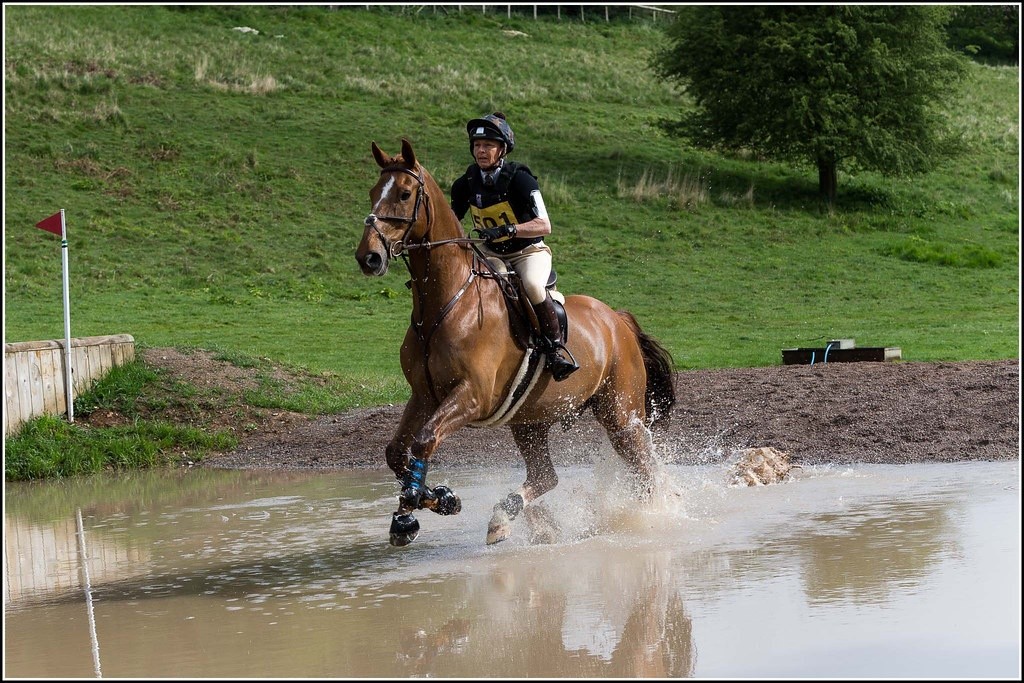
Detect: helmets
[464,113,515,160]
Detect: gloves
[479,223,518,243]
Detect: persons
[451,112,575,382]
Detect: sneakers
[547,345,578,378]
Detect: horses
[354,138,678,548]
